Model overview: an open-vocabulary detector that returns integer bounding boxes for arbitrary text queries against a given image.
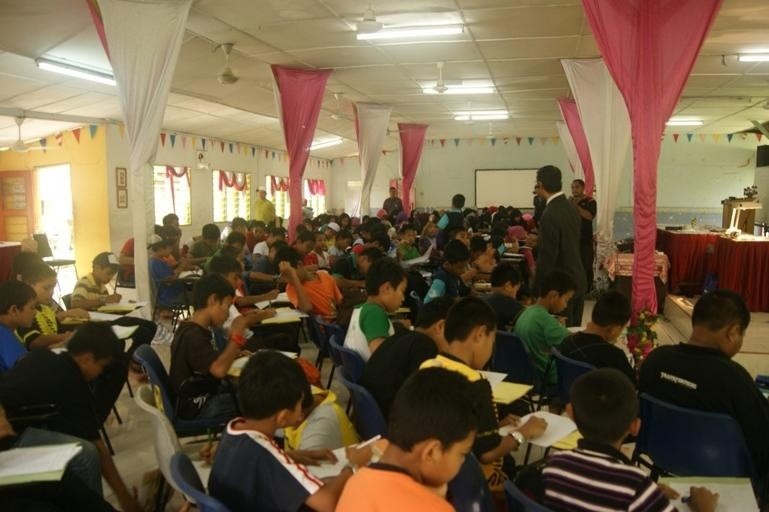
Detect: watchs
[509,430,526,447]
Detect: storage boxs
[723,198,762,233]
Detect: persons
[1,278,53,370]
[276,231,316,278]
[277,369,357,453]
[335,366,480,511]
[149,237,199,311]
[534,368,720,512]
[469,235,488,278]
[475,263,524,332]
[0,356,104,512]
[202,256,303,357]
[326,229,353,268]
[637,288,768,478]
[11,252,90,327]
[351,232,392,275]
[531,164,597,330]
[243,220,267,250]
[166,274,243,431]
[250,186,276,226]
[359,296,456,412]
[273,246,348,342]
[161,214,183,262]
[253,228,285,259]
[186,223,222,266]
[117,233,144,286]
[221,217,247,246]
[247,241,288,294]
[395,225,421,266]
[389,296,548,489]
[444,227,471,282]
[555,292,638,394]
[305,230,332,269]
[2,322,142,511]
[205,349,374,512]
[14,264,79,349]
[203,231,246,278]
[424,239,474,312]
[328,246,383,302]
[513,271,576,390]
[70,252,157,372]
[297,188,544,236]
[343,257,408,365]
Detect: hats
[93,252,127,271]
[256,186,266,192]
[147,232,177,249]
[328,222,340,234]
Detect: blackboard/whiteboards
[474,168,538,209]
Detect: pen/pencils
[113,284,118,297]
[268,298,272,308]
[356,434,381,449]
[681,496,690,503]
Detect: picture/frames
[115,167,127,188]
[117,189,129,208]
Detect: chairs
[61,293,138,398]
[112,262,135,294]
[335,364,387,443]
[501,480,551,512]
[328,334,370,426]
[31,233,79,293]
[410,291,423,310]
[137,383,214,512]
[305,309,340,370]
[410,289,425,323]
[525,342,597,464]
[637,392,758,485]
[314,313,347,389]
[489,328,553,413]
[133,343,232,458]
[152,277,191,331]
[445,447,494,512]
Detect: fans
[0,114,64,155]
[316,92,355,124]
[319,2,411,41]
[182,42,274,90]
[400,61,473,96]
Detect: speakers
[757,146,768,165]
